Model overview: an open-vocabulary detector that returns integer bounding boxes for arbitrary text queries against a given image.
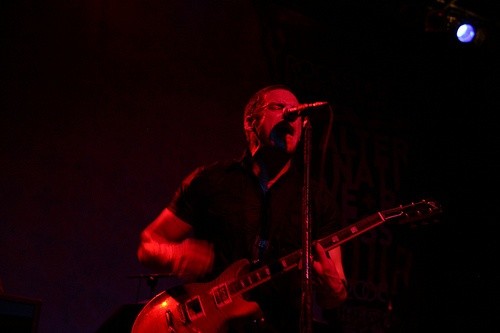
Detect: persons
[140,83,350,333]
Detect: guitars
[129,198,444,333]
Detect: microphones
[283,102,328,122]
